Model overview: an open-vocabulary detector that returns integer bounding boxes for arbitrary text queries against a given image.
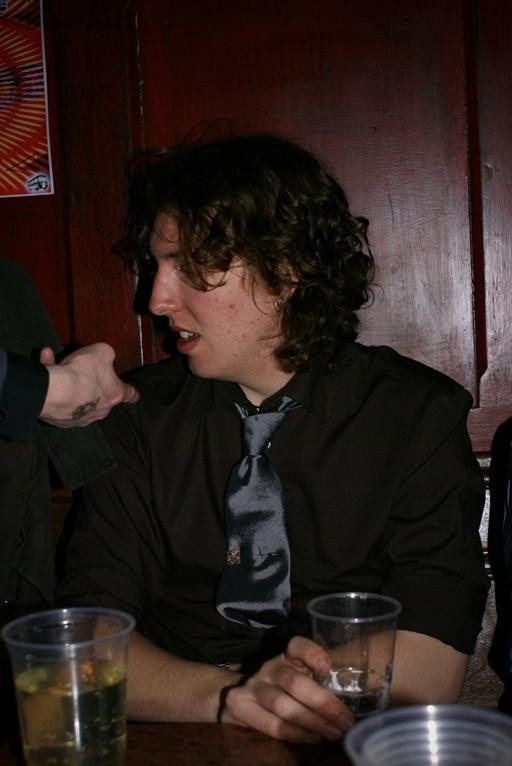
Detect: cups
[1,608,135,766]
[306,594,402,718]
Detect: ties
[213,392,309,639]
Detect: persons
[59,115,490,748]
[2,254,142,689]
